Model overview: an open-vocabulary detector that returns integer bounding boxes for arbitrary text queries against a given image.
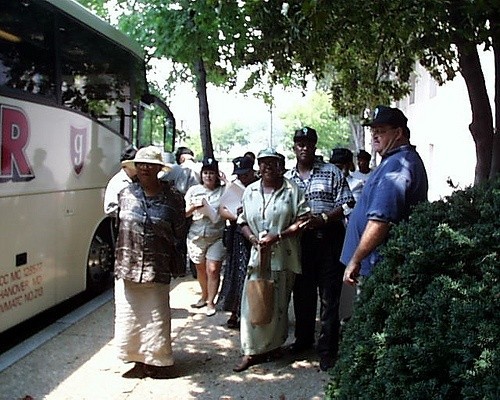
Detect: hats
[257,149,282,159]
[121,145,173,172]
[361,106,408,127]
[329,149,356,172]
[357,149,371,160]
[293,127,317,143]
[232,157,254,174]
[202,158,219,171]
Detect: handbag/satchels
[247,279,274,325]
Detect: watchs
[321,213,328,224]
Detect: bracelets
[249,235,256,240]
[278,230,286,240]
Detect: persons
[339,104,428,326]
[231,151,263,183]
[184,157,229,317]
[113,145,187,374]
[328,148,372,337]
[219,156,260,327]
[233,147,313,372]
[104,144,138,254]
[283,126,356,372]
[157,147,200,279]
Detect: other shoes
[207,309,215,315]
[289,342,307,356]
[192,299,206,308]
[235,357,255,371]
[319,350,336,372]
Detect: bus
[0,0,181,346]
